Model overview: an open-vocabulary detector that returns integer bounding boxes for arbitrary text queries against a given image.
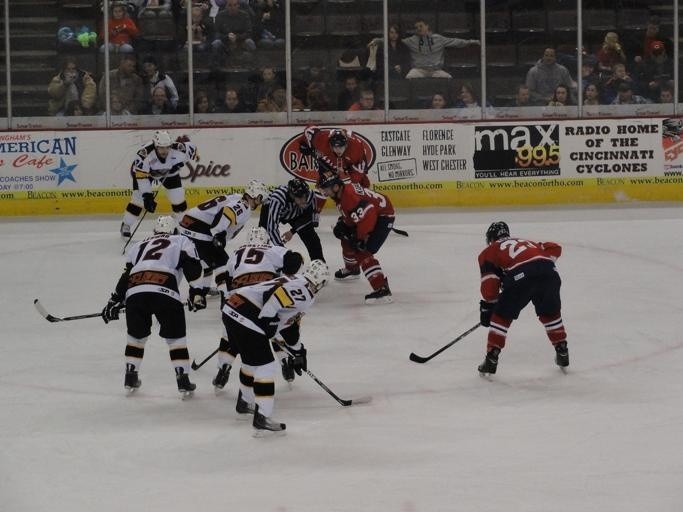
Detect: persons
[48,58,98,114]
[193,91,212,112]
[583,84,602,104]
[213,0,257,70]
[102,215,210,401]
[611,86,653,104]
[596,33,625,68]
[428,93,454,108]
[142,52,179,107]
[259,179,324,261]
[103,94,131,114]
[606,65,635,96]
[338,75,361,111]
[177,180,269,296]
[316,172,395,304]
[374,25,411,77]
[477,220,571,381]
[258,85,305,113]
[548,85,574,105]
[99,58,141,104]
[456,85,493,107]
[60,99,85,116]
[368,20,480,79]
[139,87,177,115]
[142,0,174,34]
[212,226,304,389]
[636,38,672,88]
[119,132,190,238]
[216,90,251,112]
[350,88,382,110]
[658,88,674,103]
[171,8,212,69]
[100,1,140,58]
[525,49,578,98]
[219,260,329,435]
[625,15,673,63]
[506,84,532,105]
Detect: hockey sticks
[121,169,170,255]
[191,347,220,370]
[410,322,482,363]
[33,297,187,322]
[393,229,408,237]
[274,338,372,406]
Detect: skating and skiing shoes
[124,362,143,397]
[279,358,295,390]
[119,222,132,243]
[334,266,361,281]
[363,276,396,305]
[234,388,256,421]
[212,364,232,397]
[554,340,570,375]
[173,367,197,402]
[477,347,502,378]
[251,402,287,437]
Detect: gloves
[186,286,207,313]
[478,299,495,328]
[101,292,123,324]
[289,344,308,378]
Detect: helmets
[485,221,510,244]
[153,214,179,236]
[316,170,340,188]
[244,226,270,246]
[152,130,173,149]
[243,176,270,205]
[328,129,349,147]
[287,178,310,198]
[304,259,330,294]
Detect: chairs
[293,14,326,40]
[294,47,327,73]
[556,45,589,75]
[97,53,137,82]
[443,44,481,76]
[136,51,176,80]
[582,10,618,40]
[178,83,217,109]
[98,3,136,36]
[56,4,97,50]
[486,43,518,75]
[514,10,547,39]
[135,7,176,44]
[370,76,411,106]
[330,44,368,72]
[361,13,400,39]
[519,43,557,75]
[547,10,578,40]
[217,47,255,78]
[55,55,96,83]
[217,82,256,109]
[256,48,286,75]
[486,78,517,108]
[449,79,481,103]
[517,78,526,93]
[475,10,512,39]
[177,48,216,80]
[326,12,362,37]
[437,12,475,40]
[590,44,603,53]
[410,78,449,104]
[401,12,438,38]
[617,9,651,38]
